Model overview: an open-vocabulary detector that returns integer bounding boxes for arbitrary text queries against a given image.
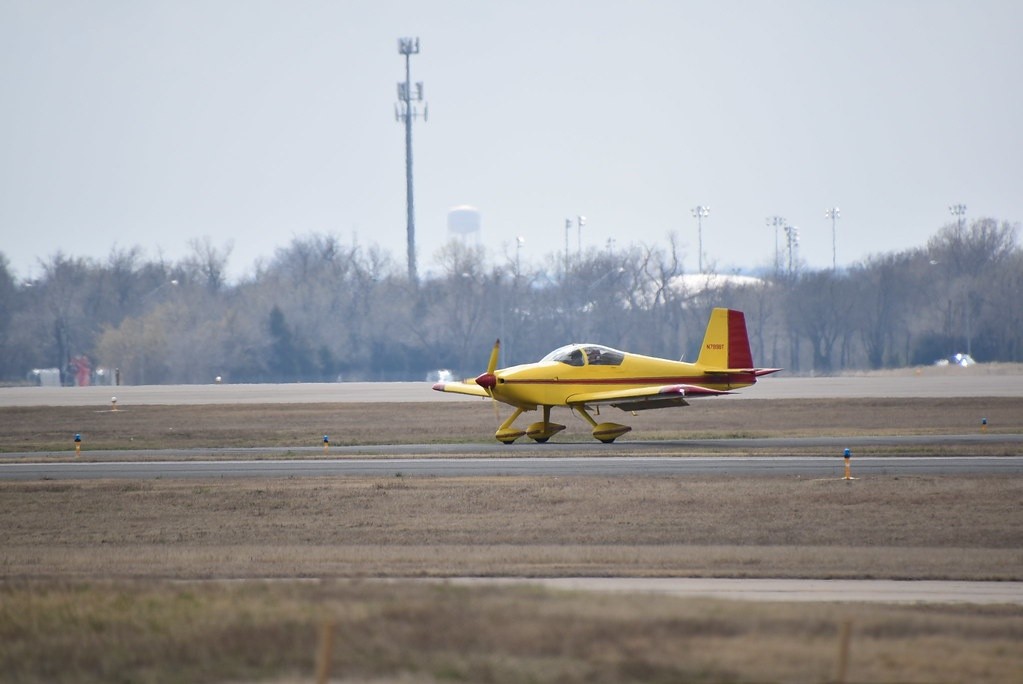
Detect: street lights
[688,205,711,274]
[510,216,583,276]
[949,203,968,240]
[767,216,798,270]
[824,208,840,268]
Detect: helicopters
[433,307,785,444]
